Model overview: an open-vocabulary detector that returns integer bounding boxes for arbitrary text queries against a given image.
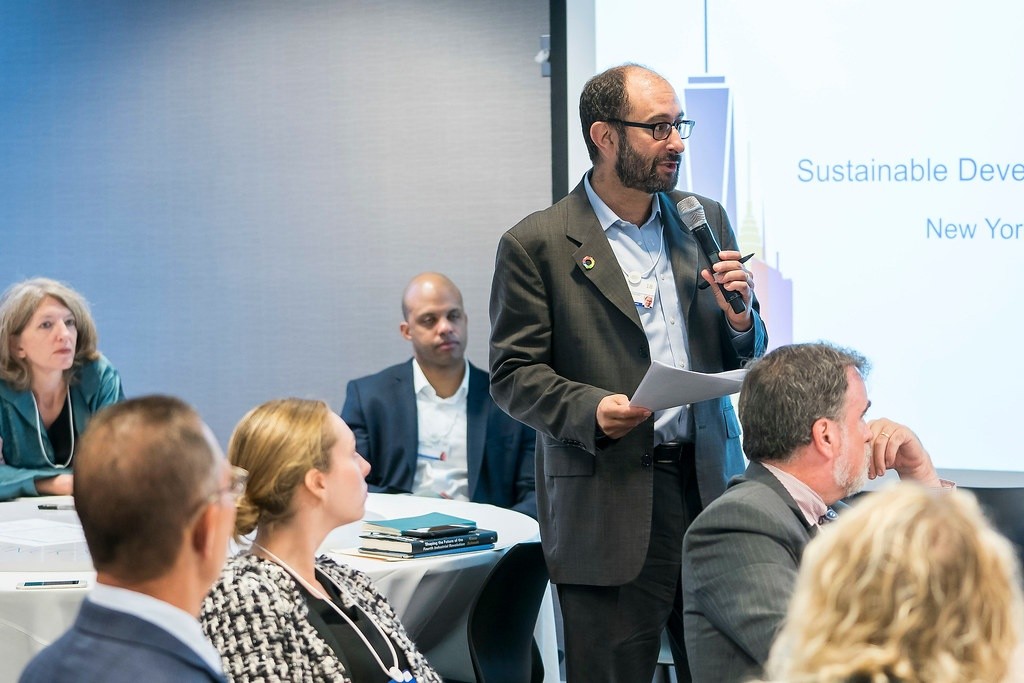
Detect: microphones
[676,196,746,314]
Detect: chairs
[466,542,552,683]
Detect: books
[358,512,498,559]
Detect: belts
[653,442,695,463]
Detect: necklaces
[31,372,74,469]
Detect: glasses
[183,467,250,526]
[597,117,695,141]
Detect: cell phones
[401,524,477,538]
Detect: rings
[880,432,891,439]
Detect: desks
[0,491,561,683]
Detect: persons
[196,397,442,683]
[488,64,770,682]
[683,344,943,683]
[742,481,1024,683]
[0,278,127,502]
[18,395,237,683]
[339,272,537,522]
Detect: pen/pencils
[38,504,74,511]
[698,252,756,291]
